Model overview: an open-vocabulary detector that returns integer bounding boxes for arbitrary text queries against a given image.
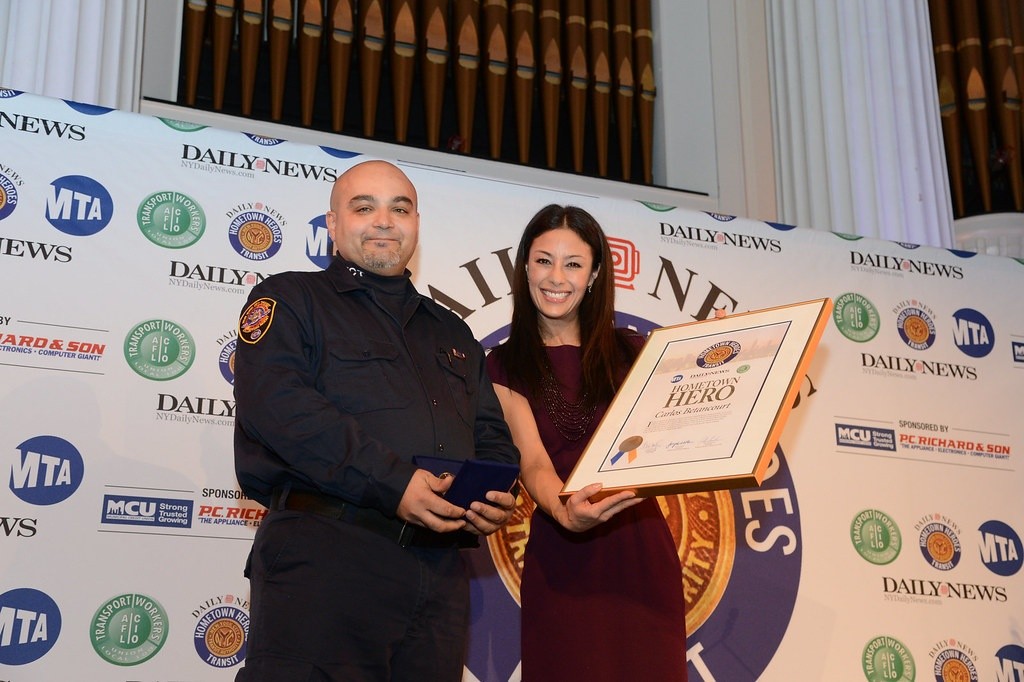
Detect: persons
[485,203,688,682]
[233,160,522,682]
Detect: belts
[269,492,459,551]
